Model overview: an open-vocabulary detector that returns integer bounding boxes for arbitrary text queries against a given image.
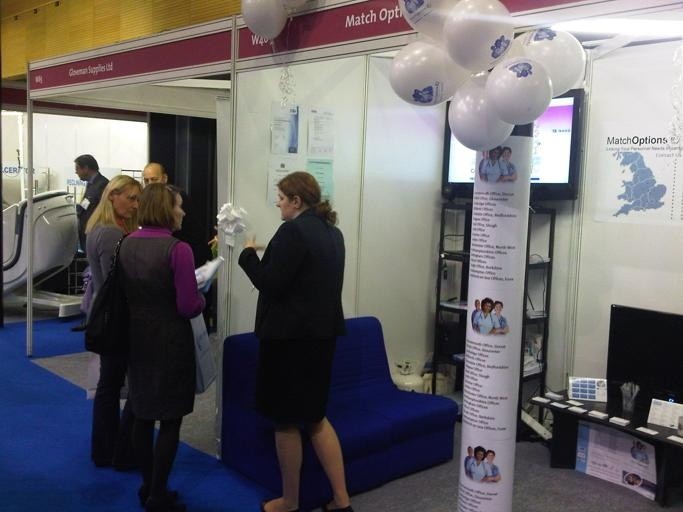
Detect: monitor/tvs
[441,88,585,200]
[606,304,683,419]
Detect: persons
[485,450,501,484]
[466,446,488,483]
[137,167,190,244]
[471,300,482,330]
[493,301,507,334]
[118,183,206,512]
[72,154,111,332]
[81,174,142,470]
[473,297,502,335]
[238,171,354,512]
[479,150,491,182]
[464,445,474,478]
[480,145,508,185]
[500,147,518,183]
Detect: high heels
[145,497,187,511]
[137,485,177,507]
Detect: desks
[528,388,683,511]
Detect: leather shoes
[321,502,354,512]
[260,496,301,512]
[72,324,85,331]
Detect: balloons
[397,0,457,41]
[387,40,471,106]
[446,0,515,71]
[448,75,515,150]
[485,58,552,125]
[507,30,586,98]
[240,0,286,42]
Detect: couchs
[220,315,459,511]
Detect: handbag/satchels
[189,312,219,394]
[85,268,126,361]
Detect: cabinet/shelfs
[431,201,557,442]
